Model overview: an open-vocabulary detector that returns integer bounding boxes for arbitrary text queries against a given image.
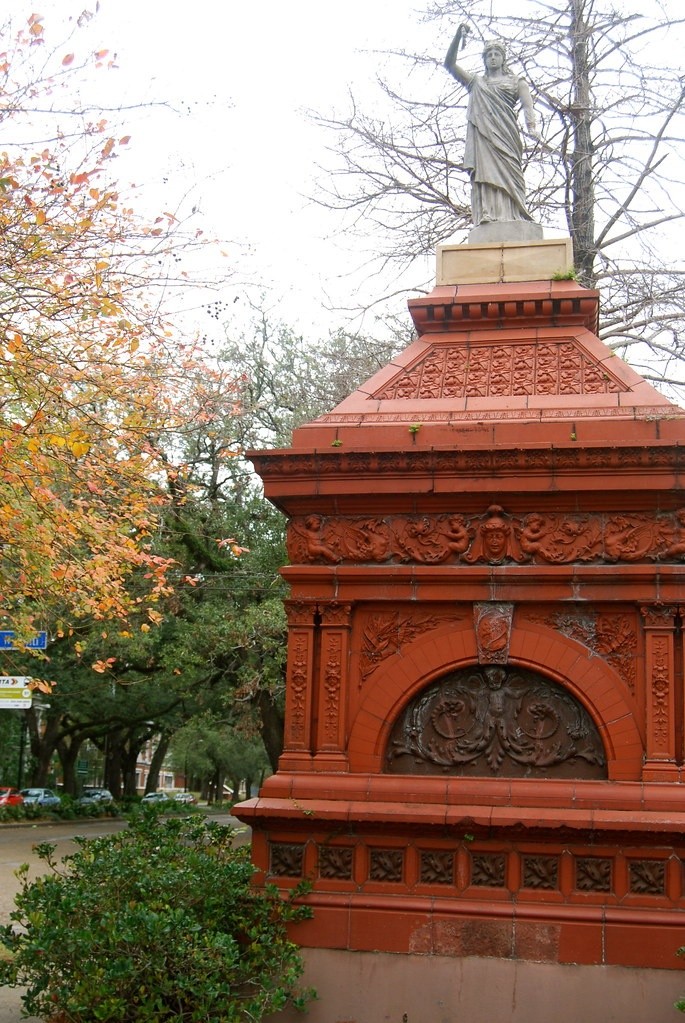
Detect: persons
[659,508,685,559]
[461,666,529,752]
[481,504,511,563]
[520,513,554,557]
[443,22,542,230]
[441,515,469,553]
[304,513,341,563]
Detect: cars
[0,787,24,807]
[142,792,170,805]
[20,788,61,809]
[174,793,198,805]
[80,789,113,806]
